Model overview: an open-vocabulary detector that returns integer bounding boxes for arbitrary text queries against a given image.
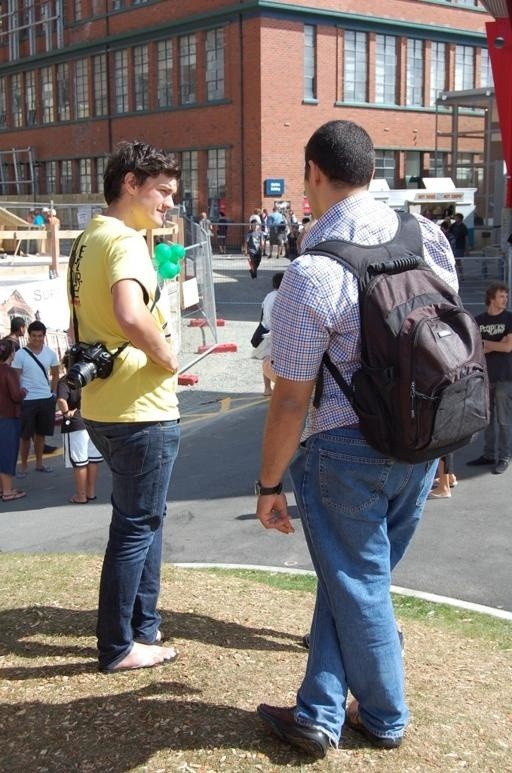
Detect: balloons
[154,242,186,279]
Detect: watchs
[254,481,282,497]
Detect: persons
[27,207,56,229]
[65,138,180,672]
[154,235,161,245]
[244,207,310,278]
[466,283,512,474]
[254,118,463,760]
[0,317,105,502]
[424,208,483,256]
[429,455,457,497]
[256,272,285,395]
[199,212,229,253]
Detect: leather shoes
[346,698,406,748]
[494,459,508,474]
[256,704,329,760]
[467,455,495,466]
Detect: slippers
[426,489,452,499]
[69,495,89,504]
[2,489,27,501]
[36,466,55,472]
[434,476,458,487]
[16,471,28,478]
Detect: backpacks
[309,210,491,461]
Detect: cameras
[63,340,115,390]
